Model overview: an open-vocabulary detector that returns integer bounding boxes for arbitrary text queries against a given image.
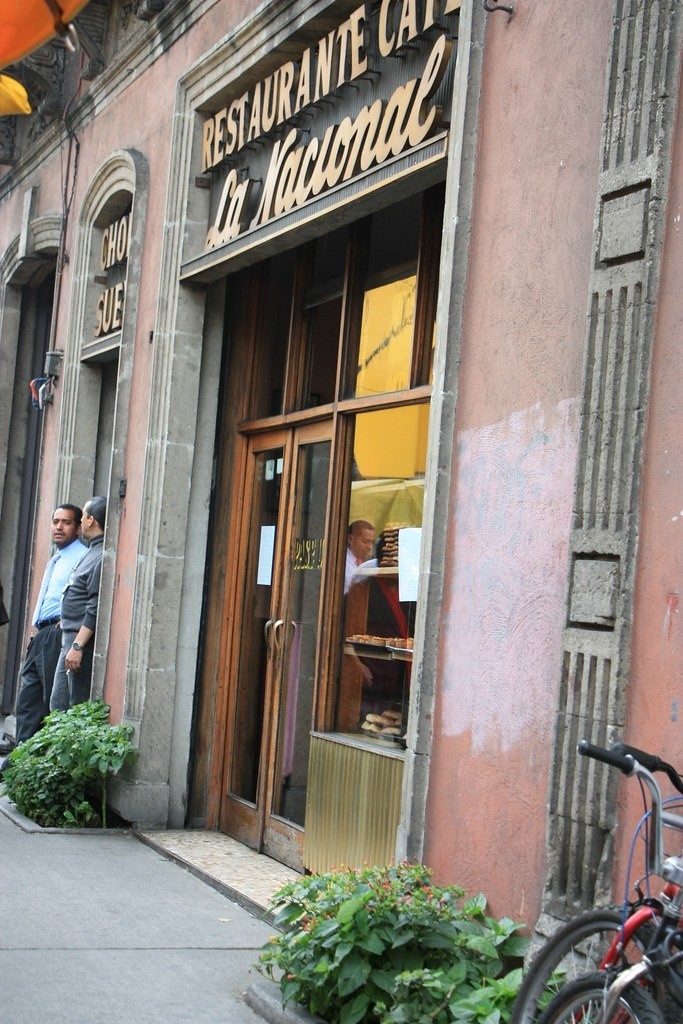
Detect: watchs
[72,642,85,651]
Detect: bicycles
[510,735,683,1023]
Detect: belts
[37,615,60,629]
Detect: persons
[343,519,376,596]
[0,502,89,782]
[348,529,409,721]
[49,496,107,714]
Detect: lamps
[137,0,164,21]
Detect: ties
[30,553,61,627]
[356,559,362,566]
